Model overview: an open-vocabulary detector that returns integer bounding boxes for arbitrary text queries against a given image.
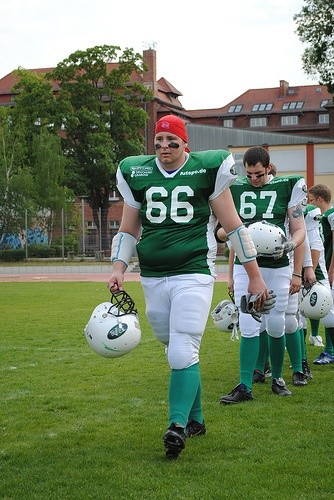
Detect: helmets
[247,219,285,258]
[211,300,239,332]
[299,284,333,320]
[83,301,142,359]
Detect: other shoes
[313,352,334,364]
[309,334,325,347]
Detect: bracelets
[293,273,302,277]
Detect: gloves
[272,234,297,260]
[240,289,277,323]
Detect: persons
[107,115,270,457]
[215,146,307,405]
[252,164,334,384]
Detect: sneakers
[264,362,271,377]
[182,419,206,437]
[302,358,313,380]
[292,371,308,387]
[163,421,185,461]
[271,377,292,396]
[252,369,265,383]
[219,382,253,404]
